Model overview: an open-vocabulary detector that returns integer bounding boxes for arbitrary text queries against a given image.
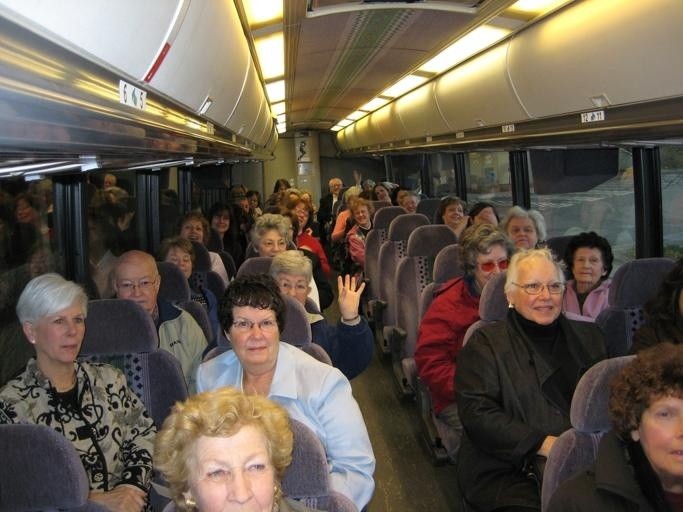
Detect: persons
[0,170,683,512]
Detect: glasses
[511,281,565,296]
[481,259,509,271]
[233,318,276,331]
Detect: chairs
[0,194,683,512]
